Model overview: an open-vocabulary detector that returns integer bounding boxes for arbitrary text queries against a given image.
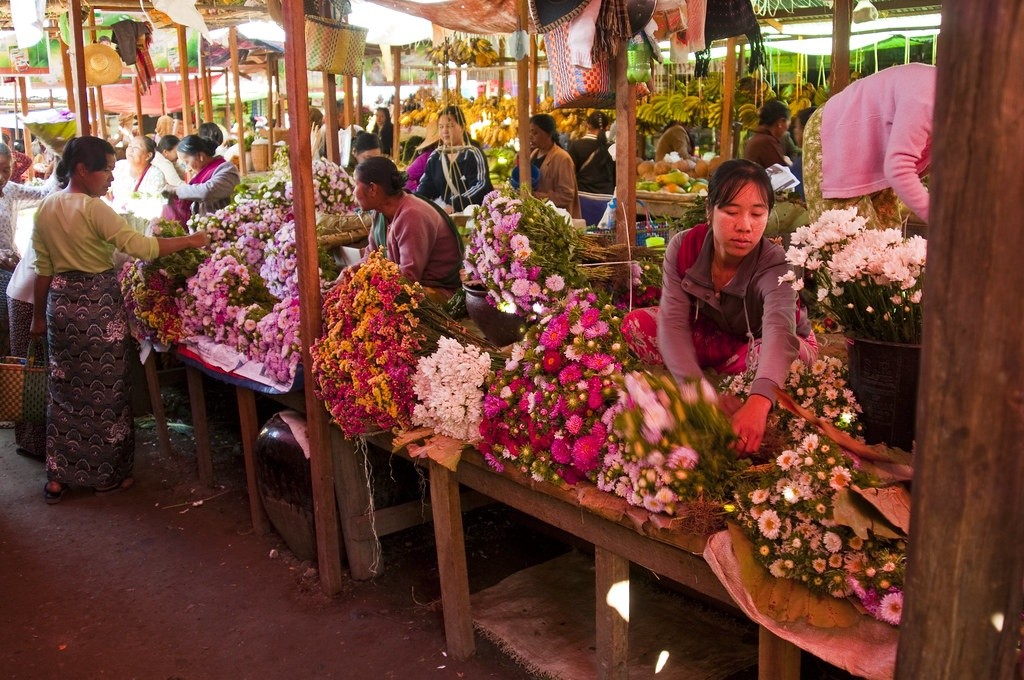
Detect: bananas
[399,95,615,146]
[426,39,498,67]
[635,76,828,136]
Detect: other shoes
[16,448,46,463]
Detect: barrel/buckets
[510,164,540,190]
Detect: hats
[84,44,122,86]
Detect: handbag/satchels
[304,0,369,80]
[543,11,651,109]
[0,341,52,422]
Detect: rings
[740,436,747,442]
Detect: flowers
[120,146,926,626]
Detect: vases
[843,327,920,456]
[460,280,537,347]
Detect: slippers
[95,477,135,497]
[44,481,68,504]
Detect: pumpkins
[635,156,721,177]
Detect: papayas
[635,170,711,192]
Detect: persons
[98,112,241,237]
[30,136,207,505]
[404,105,494,214]
[655,119,694,162]
[745,102,817,196]
[620,160,819,459]
[337,156,462,285]
[802,63,938,233]
[529,111,616,218]
[0,133,63,457]
[309,102,394,173]
